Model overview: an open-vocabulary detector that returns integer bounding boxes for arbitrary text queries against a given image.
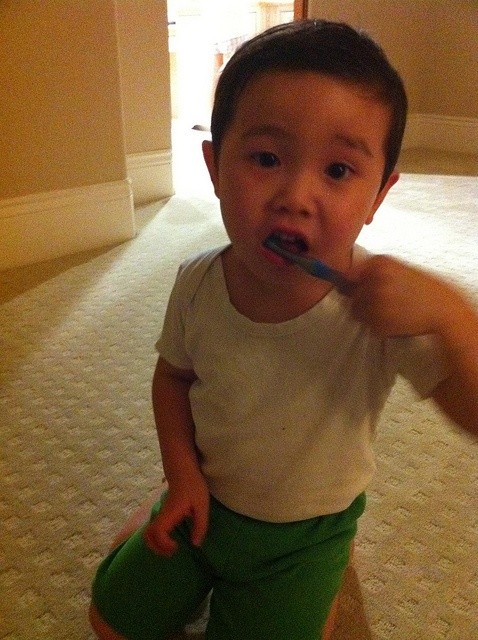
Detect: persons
[89,19,477,638]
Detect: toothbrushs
[263,237,358,297]
